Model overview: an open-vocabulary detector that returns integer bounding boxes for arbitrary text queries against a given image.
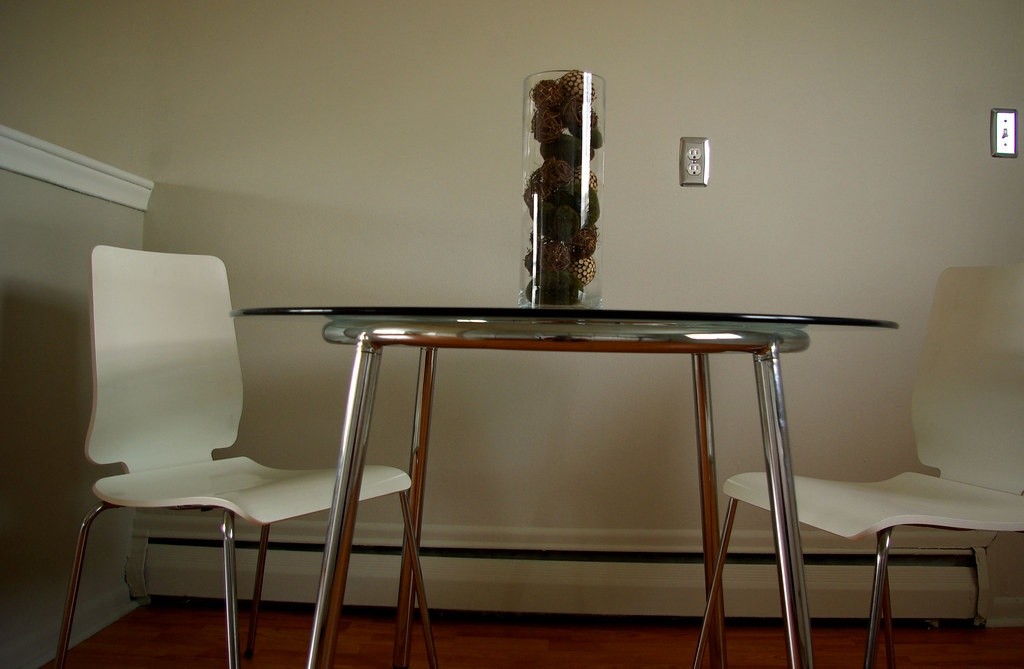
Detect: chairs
[56,246,435,669]
[694,264,1024,669]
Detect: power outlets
[679,137,710,186]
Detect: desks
[234,309,901,669]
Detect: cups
[517,69,606,308]
